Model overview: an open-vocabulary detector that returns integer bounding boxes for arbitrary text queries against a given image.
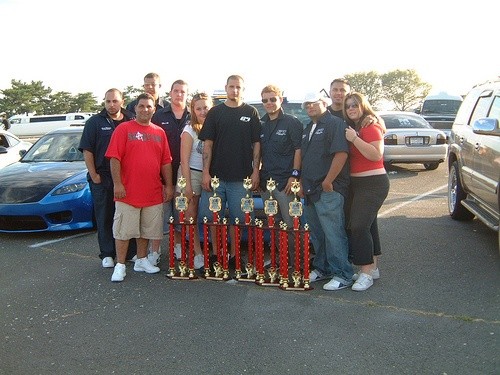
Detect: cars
[208,90,313,131]
[0,111,97,236]
[375,111,449,171]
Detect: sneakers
[352,268,380,280]
[111,263,126,282]
[128,254,137,261]
[351,273,374,291]
[302,269,331,284]
[323,278,353,291]
[133,257,160,274]
[102,256,114,267]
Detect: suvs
[445,75,500,235]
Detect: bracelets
[352,135,358,143]
[289,169,301,178]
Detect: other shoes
[148,252,161,266]
[174,243,187,260]
[193,254,205,269]
[199,247,315,274]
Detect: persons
[197,74,262,272]
[298,91,353,290]
[78,88,137,267]
[104,93,173,282]
[151,80,191,265]
[326,78,352,118]
[259,85,303,273]
[176,93,215,269]
[343,91,390,291]
[126,72,170,114]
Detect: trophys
[279,180,314,291]
[201,175,231,281]
[254,178,284,286]
[232,176,260,282]
[163,175,198,280]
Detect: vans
[414,93,464,140]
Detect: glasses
[304,101,323,110]
[261,97,280,104]
[345,101,360,110]
